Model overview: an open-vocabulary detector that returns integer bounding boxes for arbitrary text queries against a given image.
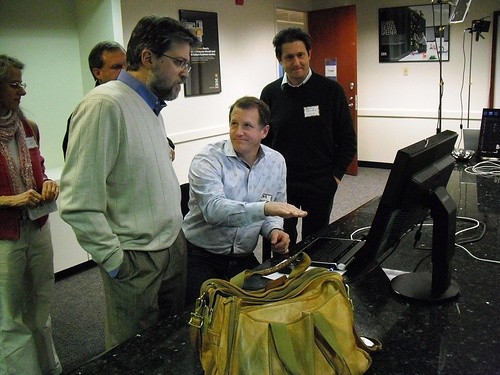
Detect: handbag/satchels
[189,251,382,375]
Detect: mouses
[278,266,292,274]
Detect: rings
[34,198,37,202]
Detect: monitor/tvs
[477,108,500,158]
[362,130,459,304]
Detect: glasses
[152,50,192,73]
[6,81,26,90]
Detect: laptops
[462,129,480,150]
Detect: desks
[60,147,500,375]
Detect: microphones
[458,124,463,148]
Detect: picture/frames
[378,3,451,62]
[178,9,221,96]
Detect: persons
[260,27,356,256]
[183,96,307,301]
[62,41,175,161]
[60,15,189,351]
[416,31,427,53]
[0,53,63,375]
